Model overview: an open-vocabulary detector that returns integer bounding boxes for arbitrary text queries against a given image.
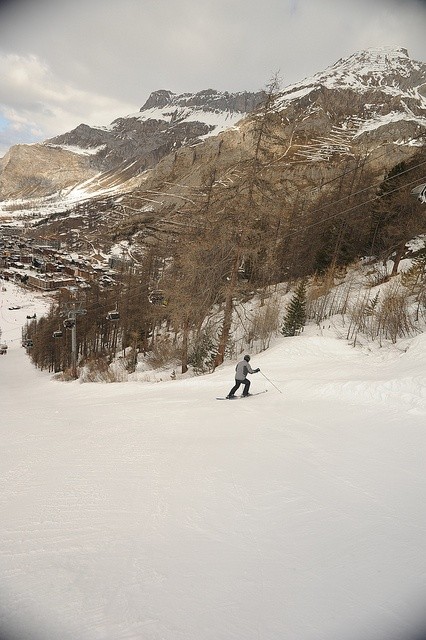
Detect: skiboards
[216,390,268,400]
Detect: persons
[226,354,260,398]
